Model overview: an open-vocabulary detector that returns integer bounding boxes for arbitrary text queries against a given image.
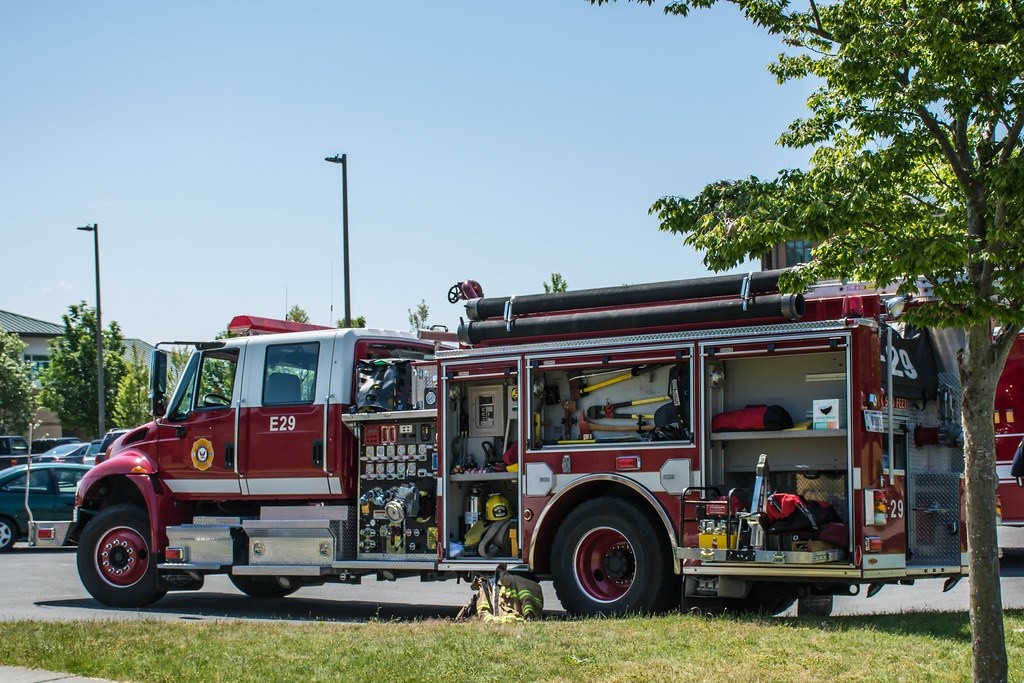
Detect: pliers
[587,395,671,418]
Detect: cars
[0,427,133,471]
[0,463,98,553]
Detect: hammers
[579,411,655,440]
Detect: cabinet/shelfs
[445,371,523,560]
[701,351,853,569]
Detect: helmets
[485,496,512,520]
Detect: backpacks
[767,492,841,535]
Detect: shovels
[570,361,666,400]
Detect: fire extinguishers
[462,488,483,542]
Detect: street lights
[77,223,107,439]
[324,153,352,329]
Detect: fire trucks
[29,265,967,620]
[958,327,1024,557]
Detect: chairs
[264,372,301,402]
